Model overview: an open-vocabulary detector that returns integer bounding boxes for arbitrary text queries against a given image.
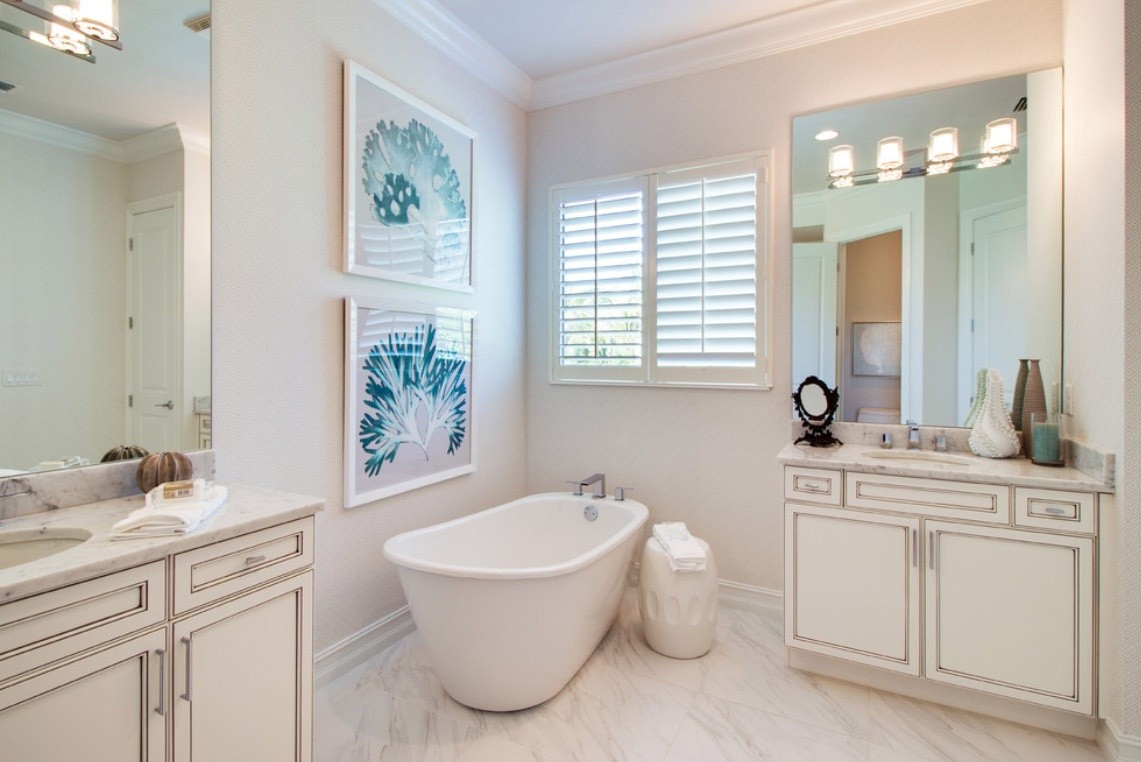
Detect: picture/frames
[851,321,902,377]
[341,60,476,294]
[344,297,478,509]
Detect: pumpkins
[137,451,194,494]
[100,444,150,464]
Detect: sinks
[862,447,979,468]
[0,526,93,571]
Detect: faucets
[904,420,923,449]
[580,473,606,498]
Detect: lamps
[827,135,1016,189]
[3,1,123,51]
[925,116,1019,164]
[823,137,905,182]
[0,2,96,64]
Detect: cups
[1031,412,1062,466]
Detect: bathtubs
[381,492,651,715]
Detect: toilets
[857,406,900,424]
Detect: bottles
[964,368,988,428]
[1010,359,1028,431]
[968,368,1020,458]
[1022,359,1046,459]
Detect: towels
[109,485,228,542]
[653,521,707,573]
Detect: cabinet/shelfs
[0,515,315,762]
[779,462,1101,741]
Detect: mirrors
[0,0,213,485]
[788,64,1065,429]
